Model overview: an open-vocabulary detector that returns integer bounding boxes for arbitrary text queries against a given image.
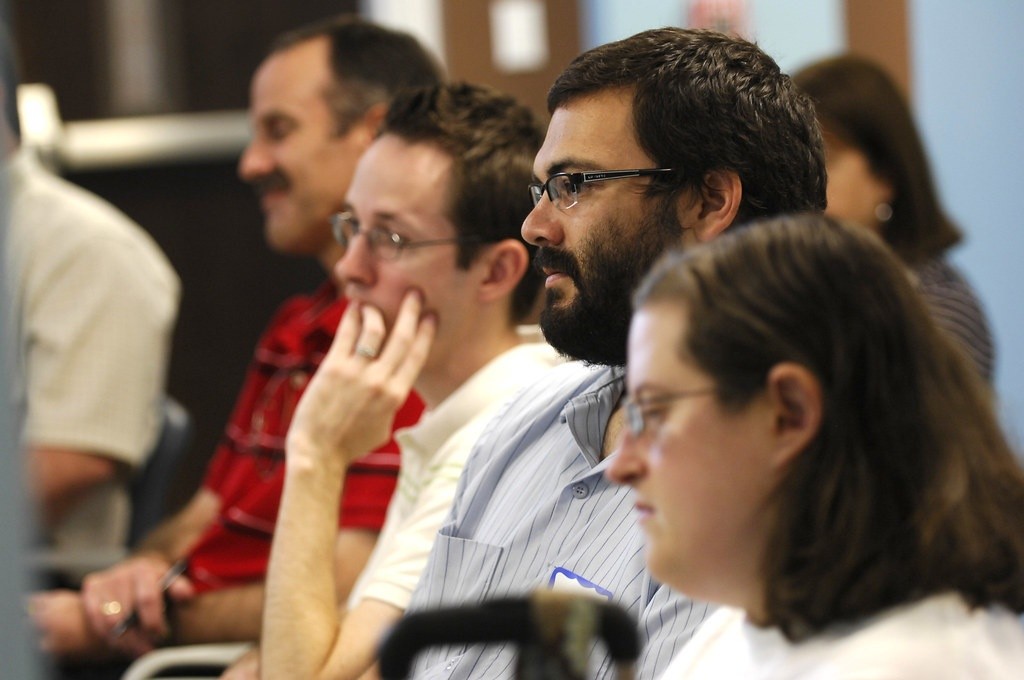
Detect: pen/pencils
[108,558,189,638]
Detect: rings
[101,601,122,615]
[355,345,378,359]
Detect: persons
[16,17,444,679]
[0,17,186,680]
[355,24,831,680]
[219,83,591,680]
[603,210,1024,679]
[791,51,998,408]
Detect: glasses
[327,211,480,258]
[526,167,695,211]
[623,384,742,437]
